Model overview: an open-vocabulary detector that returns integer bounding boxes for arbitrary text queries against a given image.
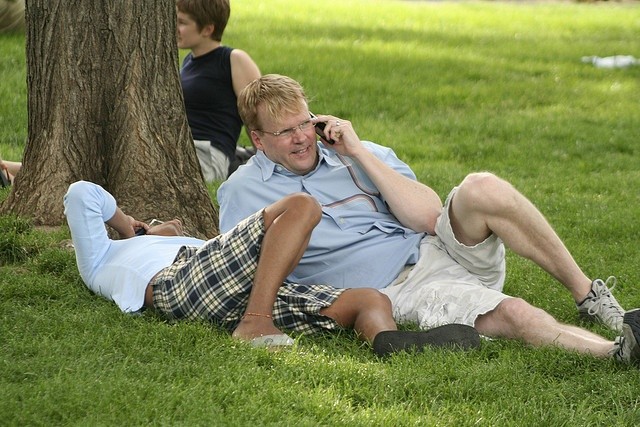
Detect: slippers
[230,333,309,353]
[373,324,483,360]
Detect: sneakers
[576,276,626,332]
[608,308,640,368]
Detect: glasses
[148,218,192,238]
[253,111,318,137]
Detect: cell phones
[308,111,334,144]
[134,222,146,236]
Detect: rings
[336,121,340,126]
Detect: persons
[215,72,639,366]
[61,180,483,360]
[1,0,261,189]
